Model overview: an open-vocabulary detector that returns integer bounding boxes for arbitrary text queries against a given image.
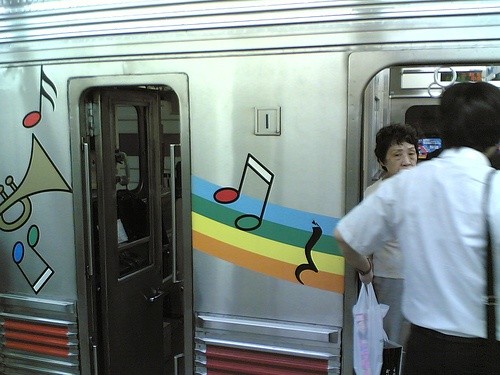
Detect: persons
[333,80,500,375]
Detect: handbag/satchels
[352,282,390,375]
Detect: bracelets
[358,257,371,276]
